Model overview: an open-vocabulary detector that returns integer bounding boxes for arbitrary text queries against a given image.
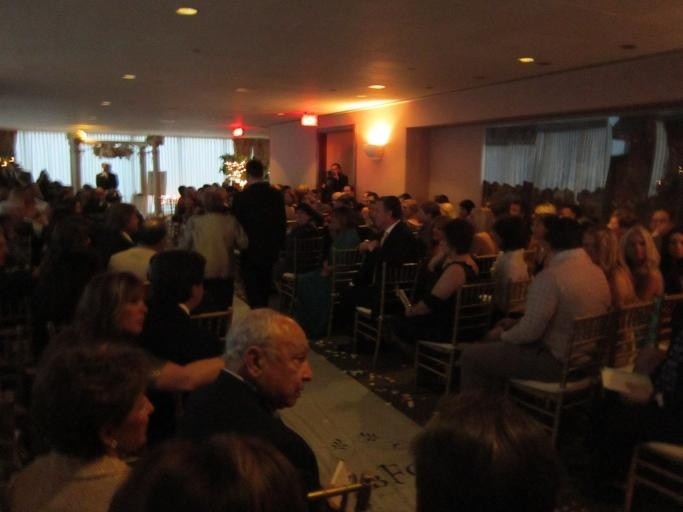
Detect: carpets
[221,293,424,512]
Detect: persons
[1,149,682,512]
